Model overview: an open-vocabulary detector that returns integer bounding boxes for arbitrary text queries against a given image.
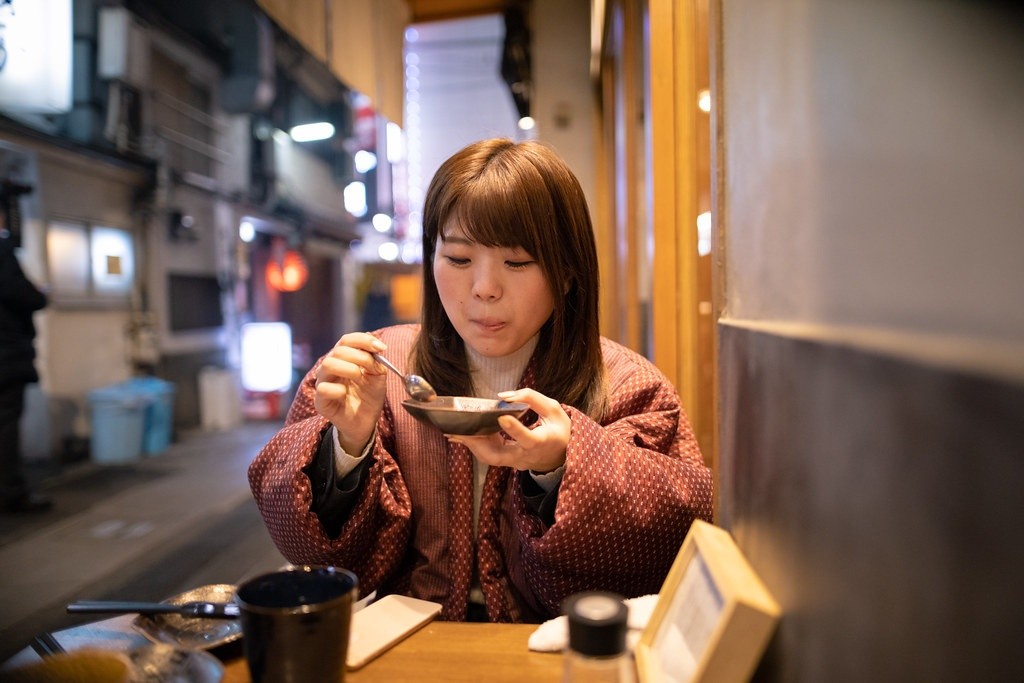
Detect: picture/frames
[633,518,782,682]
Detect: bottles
[562,593,638,683]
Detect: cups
[234,566,358,683]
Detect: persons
[247,138,714,622]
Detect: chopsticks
[30,632,67,661]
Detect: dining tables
[205,623,644,683]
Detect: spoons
[371,352,437,402]
[66,600,240,619]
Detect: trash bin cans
[85,376,178,467]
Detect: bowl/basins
[132,584,242,650]
[401,396,530,435]
